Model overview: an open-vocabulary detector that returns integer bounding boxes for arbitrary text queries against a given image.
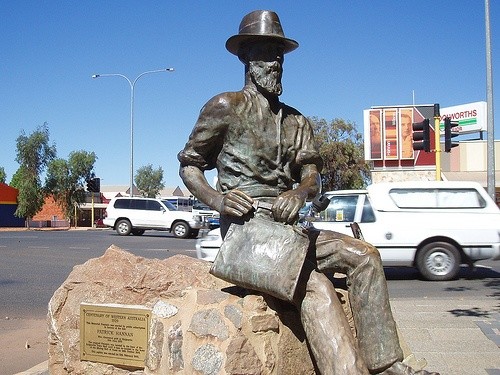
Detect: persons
[178,11,440,375]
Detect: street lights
[92,68,173,199]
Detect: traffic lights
[445,117,459,153]
[412,118,430,152]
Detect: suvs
[102,195,207,239]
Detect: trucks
[193,181,500,284]
[192,196,220,229]
